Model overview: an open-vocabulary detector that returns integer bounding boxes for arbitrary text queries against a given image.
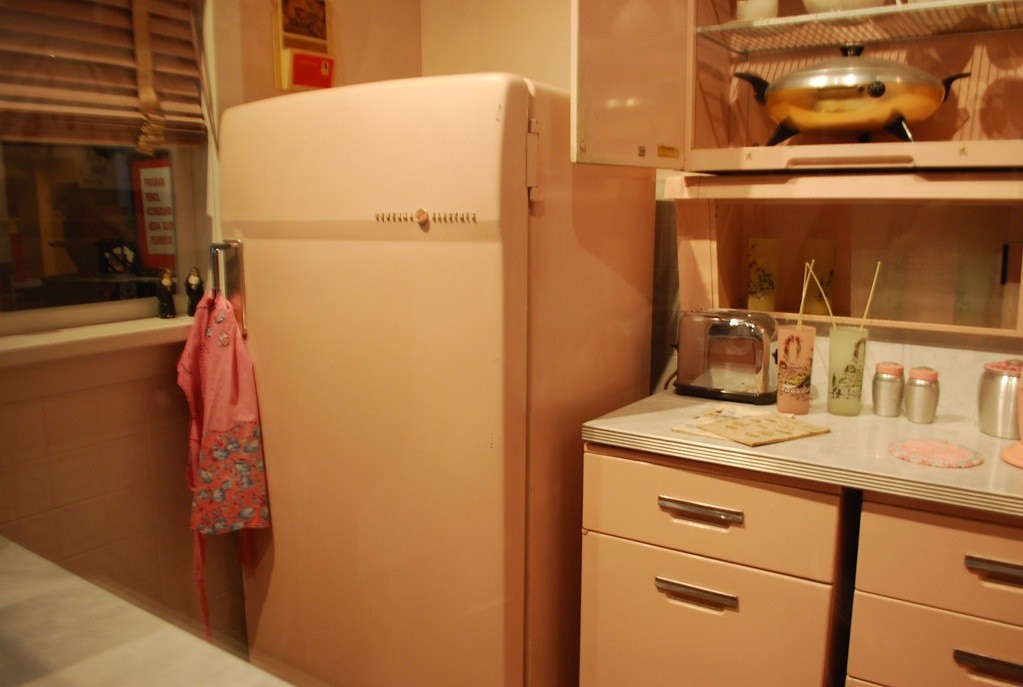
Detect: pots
[733,42,971,143]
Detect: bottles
[159,268,177,318]
[906,363,939,426]
[185,267,203,316]
[873,362,906,419]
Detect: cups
[975,359,1023,440]
[776,324,817,412]
[825,322,869,414]
[736,0,778,20]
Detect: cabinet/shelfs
[844,490,1023,687]
[577,444,862,687]
[569,0,1023,340]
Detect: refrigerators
[217,70,653,687]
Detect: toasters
[673,307,777,405]
